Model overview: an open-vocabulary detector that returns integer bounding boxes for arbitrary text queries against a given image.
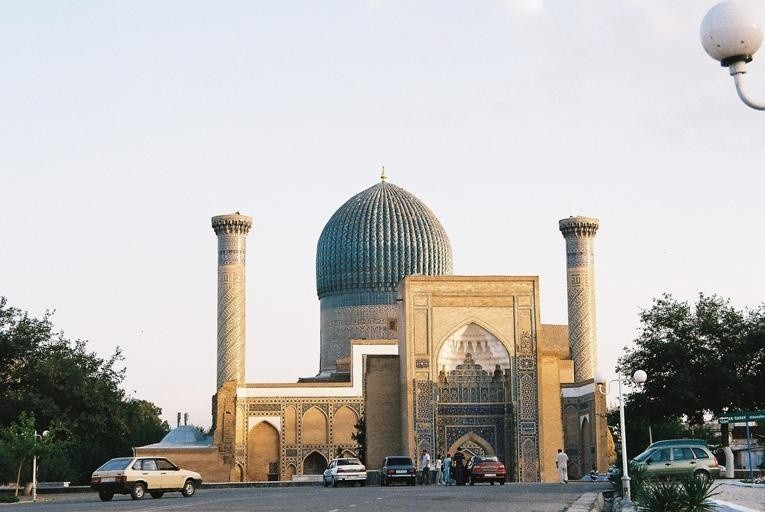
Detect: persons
[420,447,481,486]
[556,448,569,484]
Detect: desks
[19,430,50,501]
[593,365,648,501]
[699,0,765,111]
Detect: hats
[91,456,202,501]
[381,456,417,486]
[323,458,368,487]
[464,455,507,485]
[629,438,726,484]
